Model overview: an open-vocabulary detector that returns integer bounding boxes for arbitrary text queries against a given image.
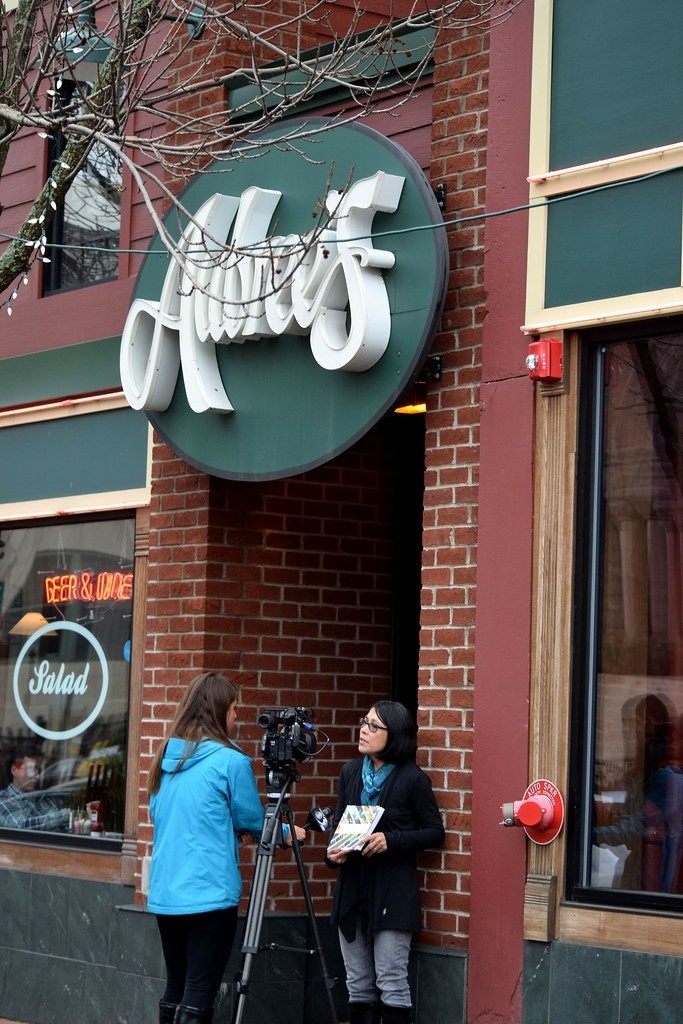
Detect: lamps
[8,612,58,636]
[48,0,207,64]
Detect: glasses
[360,717,389,733]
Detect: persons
[324,701,446,1024]
[594,694,683,894]
[147,672,306,1024]
[0,747,85,833]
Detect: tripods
[230,760,339,1024]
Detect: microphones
[302,805,335,833]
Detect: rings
[374,847,377,850]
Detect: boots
[159,999,179,1024]
[348,1001,414,1024]
[174,1005,214,1024]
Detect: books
[328,805,385,851]
